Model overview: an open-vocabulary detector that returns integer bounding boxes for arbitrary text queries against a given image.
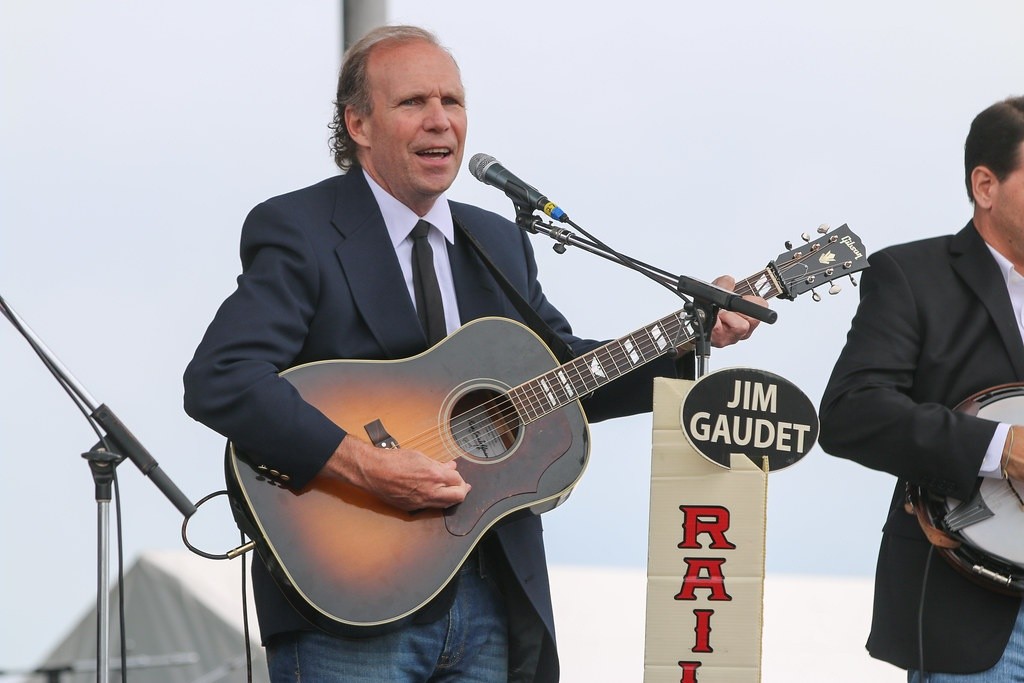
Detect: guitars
[221,220,870,629]
[903,379,1024,594]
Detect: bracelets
[1000,427,1014,479]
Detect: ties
[410,219,447,350]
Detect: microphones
[468,153,569,223]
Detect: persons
[816,93,1024,683]
[183,26,770,683]
